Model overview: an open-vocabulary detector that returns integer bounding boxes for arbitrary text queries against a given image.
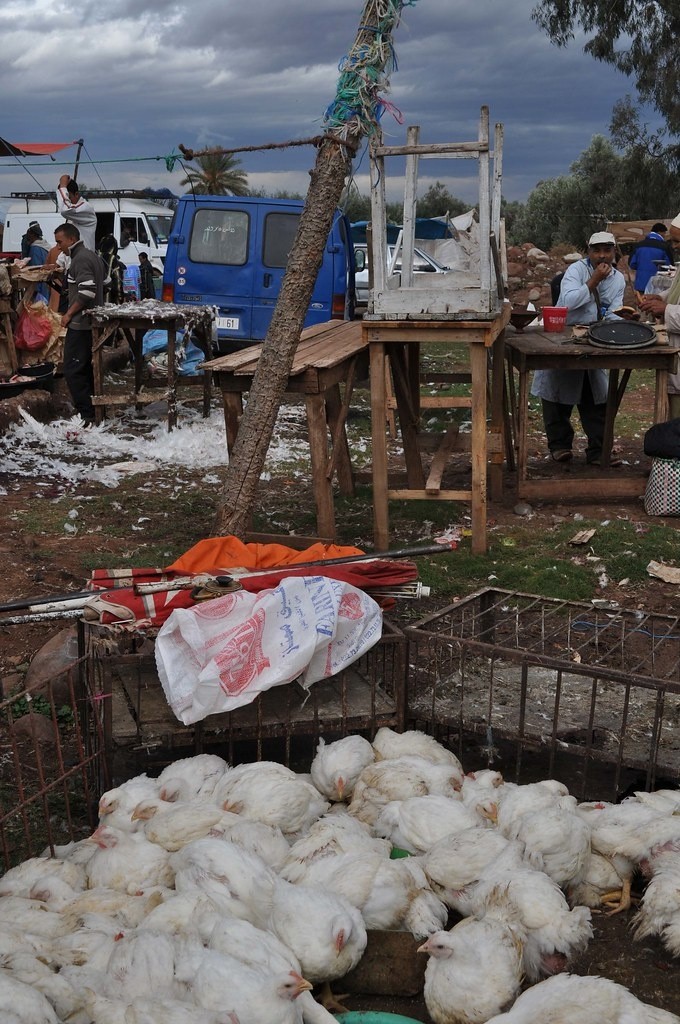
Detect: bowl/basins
[509,310,540,333]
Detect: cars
[354,244,460,304]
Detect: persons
[638,213,680,422]
[529,230,626,467]
[628,222,675,295]
[22,174,156,426]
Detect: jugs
[190,576,244,605]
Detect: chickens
[0,726,680,1024]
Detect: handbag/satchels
[643,456,680,516]
[14,302,52,351]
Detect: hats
[671,213,680,228]
[652,223,667,232]
[589,231,615,247]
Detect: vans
[1,197,174,277]
[161,193,366,350]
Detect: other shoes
[551,449,572,461]
[591,455,622,466]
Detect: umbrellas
[27,556,432,630]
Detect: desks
[194,319,369,542]
[83,303,218,434]
[360,314,513,555]
[504,328,679,501]
[367,105,508,320]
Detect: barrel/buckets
[15,361,55,395]
[541,306,568,332]
